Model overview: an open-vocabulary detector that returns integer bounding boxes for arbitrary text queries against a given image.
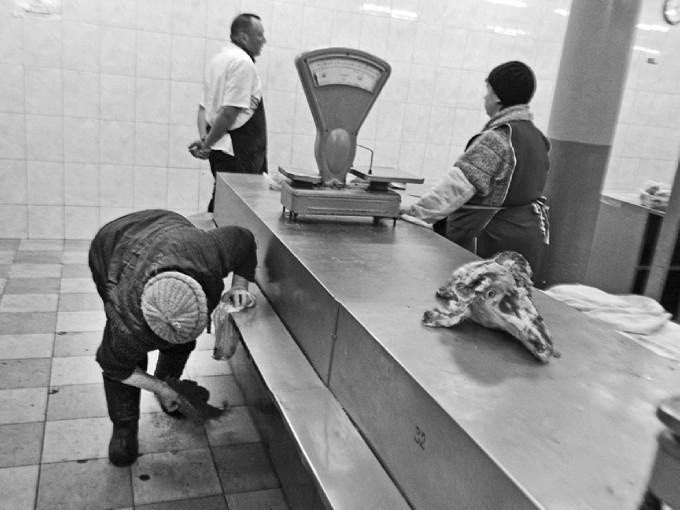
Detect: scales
[278,46,424,228]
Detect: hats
[490,61,534,105]
[142,271,209,344]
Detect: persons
[189,14,267,213]
[398,62,550,290]
[87,210,257,467]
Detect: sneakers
[110,423,139,466]
